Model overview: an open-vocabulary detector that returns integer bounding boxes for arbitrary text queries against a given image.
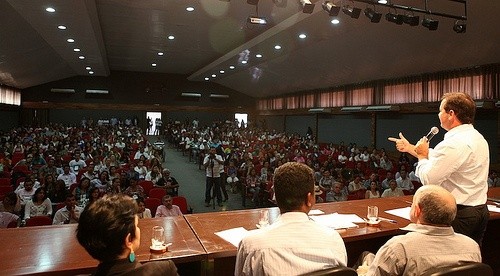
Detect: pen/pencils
[364,217,369,225]
[377,217,398,223]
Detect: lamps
[342,0,361,20]
[273,0,287,8]
[321,0,341,17]
[403,8,420,27]
[421,12,440,31]
[384,6,405,25]
[364,3,383,24]
[452,18,467,34]
[297,0,316,15]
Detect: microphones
[425,126,439,142]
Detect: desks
[395,185,500,222]
[185,195,414,276]
[0,215,209,276]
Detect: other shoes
[204,203,210,207]
[225,197,228,200]
[211,196,215,199]
[218,203,223,207]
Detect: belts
[457,204,485,210]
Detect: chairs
[0,125,421,228]
[419,261,494,276]
[296,265,358,276]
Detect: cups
[368,206,378,223]
[259,210,269,228]
[152,226,166,248]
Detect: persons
[234,162,347,276]
[356,183,482,276]
[0,192,21,214]
[388,91,489,254]
[163,117,422,208]
[136,197,152,218]
[74,194,181,276]
[23,187,53,220]
[211,147,229,202]
[0,116,180,206]
[155,195,183,218]
[0,211,21,228]
[202,147,225,208]
[52,193,85,225]
